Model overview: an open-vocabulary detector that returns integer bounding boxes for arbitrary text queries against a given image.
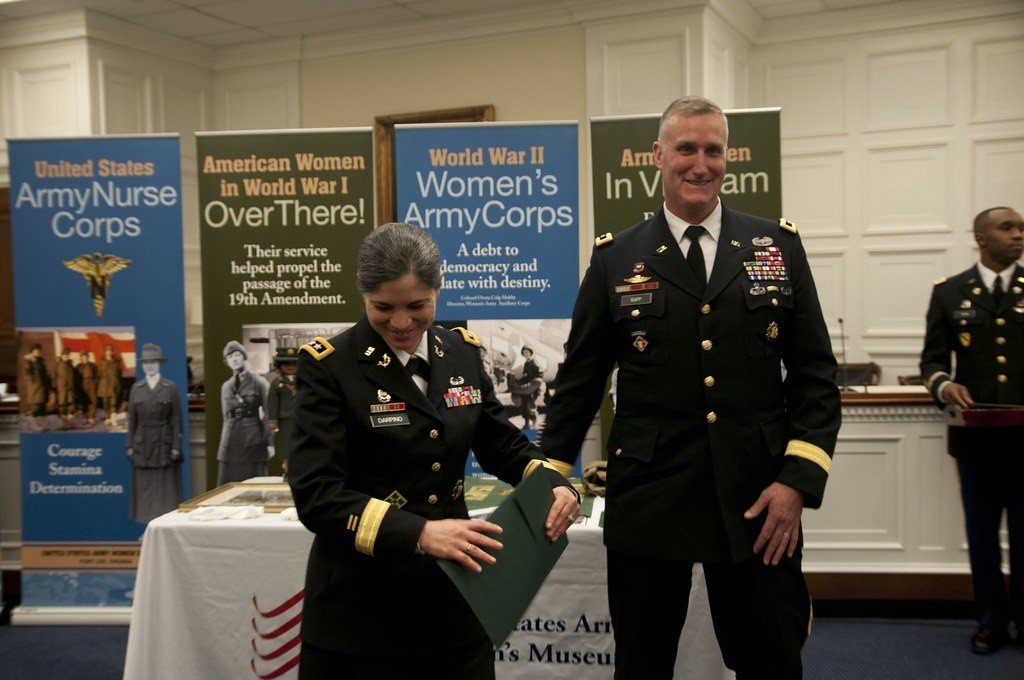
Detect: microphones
[838,319,857,393]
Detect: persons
[479,342,568,430]
[285,222,582,680]
[267,347,299,477]
[919,207,1024,654]
[126,343,185,542]
[537,98,842,680]
[187,356,194,394]
[217,340,270,487]
[21,342,122,431]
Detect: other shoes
[970,622,1011,655]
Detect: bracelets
[415,543,424,556]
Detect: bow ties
[404,357,429,380]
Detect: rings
[565,516,574,526]
[784,531,791,538]
[466,544,472,554]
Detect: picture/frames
[180,482,294,512]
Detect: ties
[992,277,1005,315]
[233,374,241,390]
[683,226,707,286]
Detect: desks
[115,475,737,680]
[790,385,1010,601]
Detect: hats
[28,342,41,351]
[272,345,300,363]
[222,340,247,359]
[102,344,114,351]
[136,343,167,364]
[60,347,71,354]
[520,344,534,356]
